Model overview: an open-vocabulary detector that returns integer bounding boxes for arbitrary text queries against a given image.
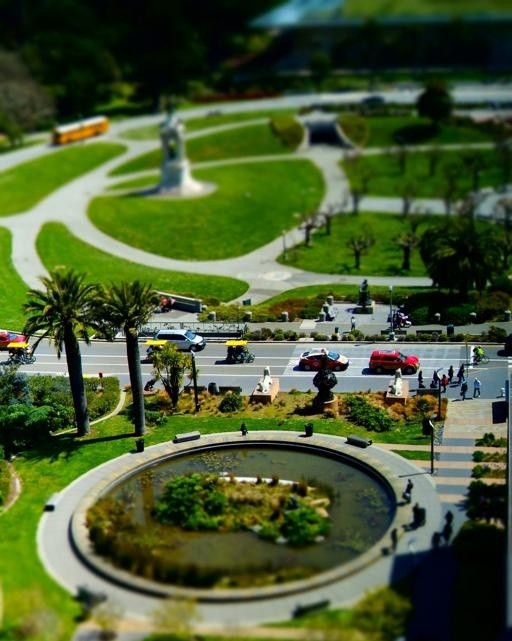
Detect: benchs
[174,430,201,443]
[347,433,372,449]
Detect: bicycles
[472,354,490,365]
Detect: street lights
[388,285,393,331]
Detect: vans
[152,329,206,351]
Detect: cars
[299,347,349,372]
[368,350,420,375]
[0,331,29,348]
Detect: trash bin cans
[447,324,454,337]
[243,298,251,305]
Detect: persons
[391,528,397,552]
[412,503,422,526]
[432,510,453,548]
[351,316,356,330]
[473,378,481,398]
[416,363,468,401]
[473,345,485,361]
[240,423,248,436]
[405,479,413,503]
[393,311,402,330]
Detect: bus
[51,115,109,146]
